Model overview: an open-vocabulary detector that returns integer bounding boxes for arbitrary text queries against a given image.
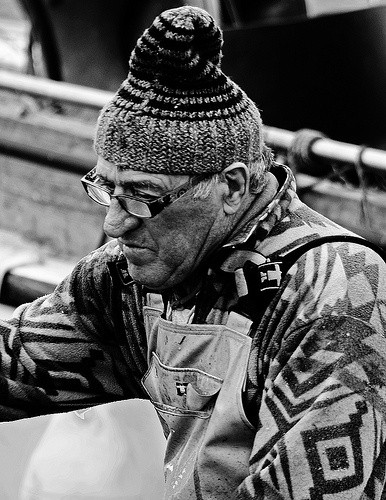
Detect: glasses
[81,165,212,218]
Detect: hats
[93,6,263,174]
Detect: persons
[0,6,386,500]
